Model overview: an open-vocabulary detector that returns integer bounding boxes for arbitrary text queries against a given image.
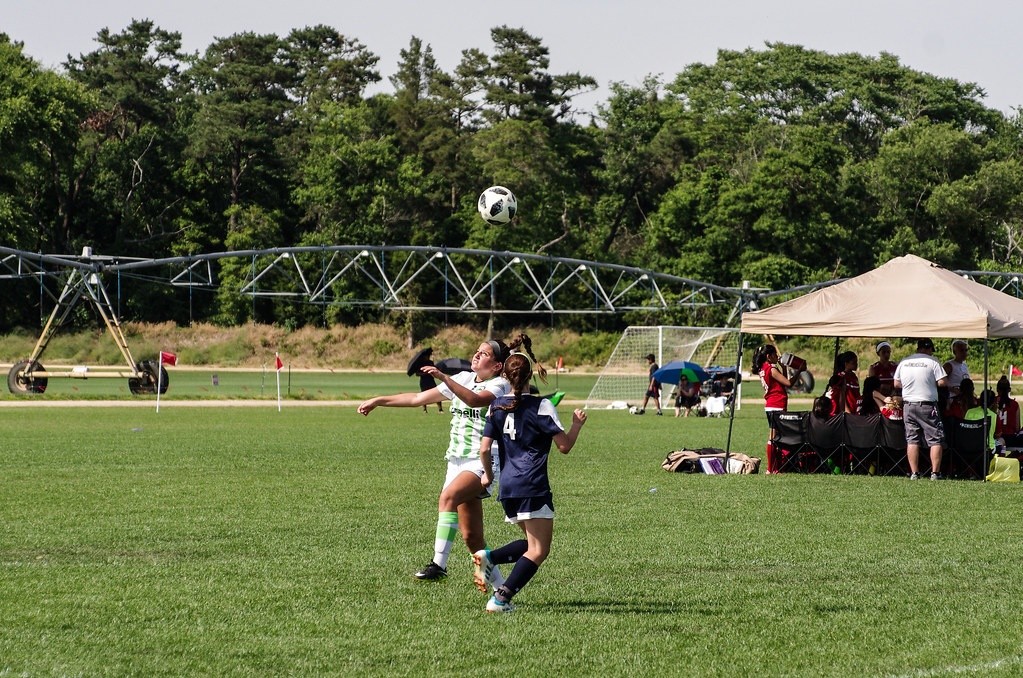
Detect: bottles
[1001,446,1006,453]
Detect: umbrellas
[436,357,472,370]
[710,371,741,386]
[407,348,432,377]
[650,361,708,404]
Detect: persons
[752,345,804,474]
[870,341,897,394]
[893,338,954,479]
[716,376,732,402]
[945,340,1020,457]
[674,375,698,417]
[416,354,443,413]
[634,354,662,415]
[872,391,903,420]
[814,351,860,419]
[357,334,548,593]
[471,353,587,612]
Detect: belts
[903,401,937,407]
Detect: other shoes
[910,473,922,480]
[438,411,445,414]
[635,409,646,415]
[931,471,946,481]
[766,469,781,474]
[656,412,662,415]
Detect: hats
[644,354,655,360]
[918,338,935,352]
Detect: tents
[724,253,1023,482]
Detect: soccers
[477,186,519,226]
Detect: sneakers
[415,559,448,581]
[472,549,494,594]
[485,595,514,614]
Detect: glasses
[682,378,687,381]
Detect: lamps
[82,246,92,256]
[87,273,98,284]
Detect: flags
[162,352,176,364]
[276,358,283,371]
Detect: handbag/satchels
[662,447,762,474]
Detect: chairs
[843,412,880,476]
[950,415,991,481]
[880,414,912,477]
[678,382,734,418]
[770,411,809,474]
[917,427,953,478]
[809,410,843,474]
